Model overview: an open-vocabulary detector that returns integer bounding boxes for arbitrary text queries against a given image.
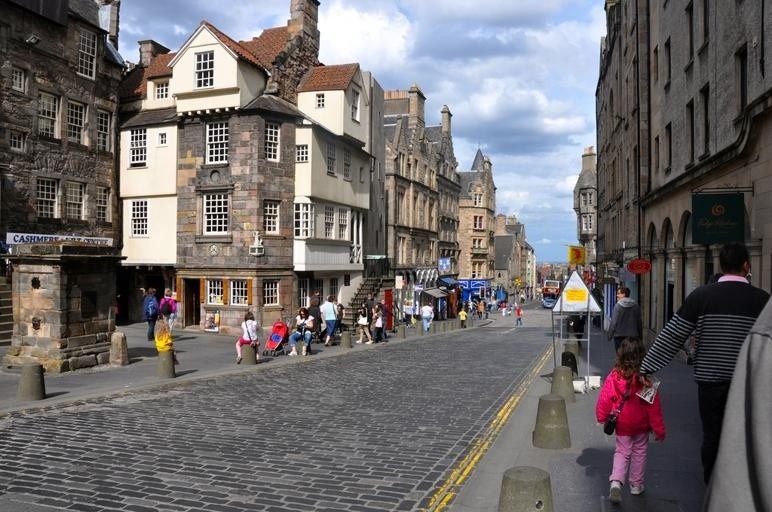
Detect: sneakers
[302,351,306,356]
[288,351,297,356]
[237,357,242,364]
[630,484,645,494]
[331,343,337,345]
[256,359,262,364]
[609,481,622,503]
[356,338,388,345]
[325,343,328,346]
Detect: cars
[544,299,557,308]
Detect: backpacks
[161,298,171,315]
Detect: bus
[543,280,563,297]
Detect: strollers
[263,319,291,356]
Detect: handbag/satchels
[250,341,260,347]
[604,414,617,435]
[358,315,369,325]
[297,323,306,331]
[147,300,156,317]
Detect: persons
[568,312,586,346]
[235,312,260,364]
[595,337,666,505]
[307,293,523,346]
[153,319,180,365]
[159,288,177,335]
[288,308,315,356]
[639,244,772,488]
[607,287,643,354]
[142,288,160,341]
